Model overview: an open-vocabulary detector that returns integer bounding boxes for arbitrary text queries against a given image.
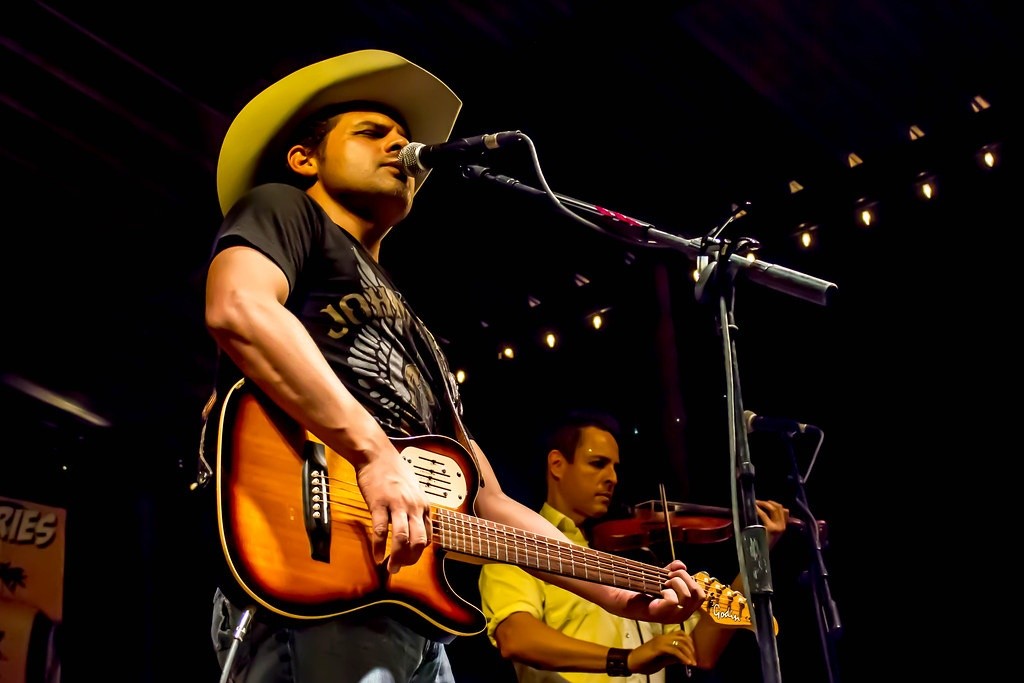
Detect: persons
[205,49,707,683]
[478,416,790,683]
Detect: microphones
[398,130,522,178]
[744,410,807,433]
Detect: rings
[672,640,678,648]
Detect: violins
[587,490,833,550]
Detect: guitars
[192,363,783,652]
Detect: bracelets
[606,647,633,677]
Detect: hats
[217,50,464,218]
